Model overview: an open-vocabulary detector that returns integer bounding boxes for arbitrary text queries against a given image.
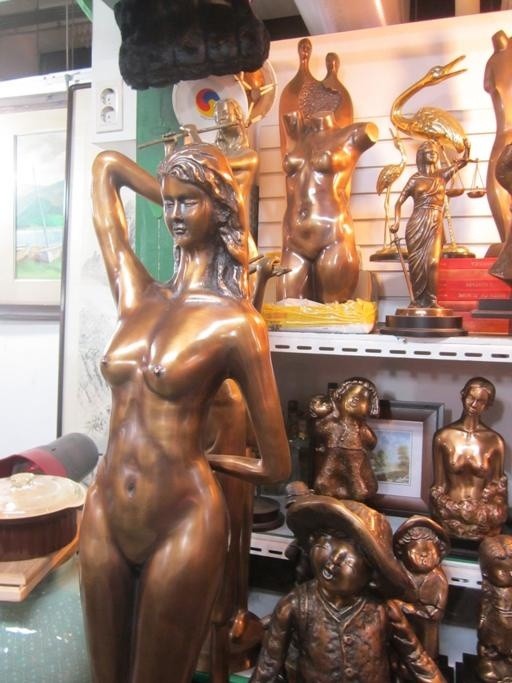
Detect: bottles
[285,382,338,486]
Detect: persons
[431,378,511,544]
[75,144,290,680]
[248,494,446,683]
[389,511,448,664]
[388,137,471,309]
[160,99,260,208]
[307,374,379,501]
[275,112,379,307]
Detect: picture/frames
[0,91,67,323]
[308,400,445,518]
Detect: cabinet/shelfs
[227,331,512,591]
